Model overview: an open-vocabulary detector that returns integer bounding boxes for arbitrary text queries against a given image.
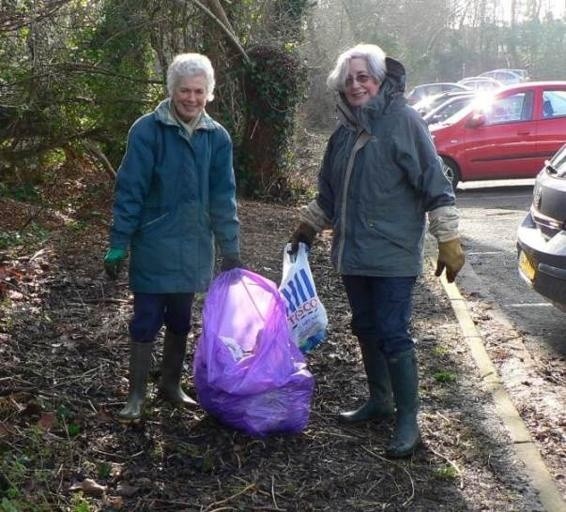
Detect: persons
[285,43,466,457]
[103,52,243,422]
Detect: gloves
[435,238,465,282]
[287,222,316,254]
[222,259,249,286]
[104,249,128,279]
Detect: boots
[158,330,198,407]
[340,335,393,424]
[119,342,154,419]
[386,349,419,457]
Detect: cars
[408,65,565,314]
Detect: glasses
[346,75,371,84]
